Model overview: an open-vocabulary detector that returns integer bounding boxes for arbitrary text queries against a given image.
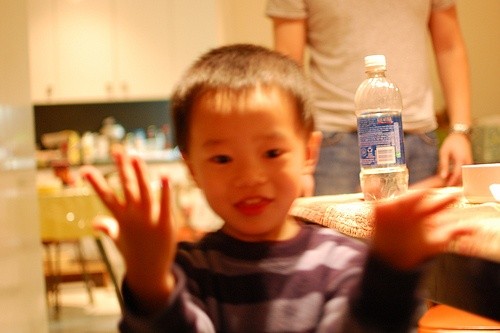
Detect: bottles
[352,52,410,203]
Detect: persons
[79,41,477,333]
[264,0,476,199]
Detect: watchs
[448,123,474,141]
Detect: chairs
[286,182,500,331]
[39,190,128,319]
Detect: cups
[461,162,500,204]
